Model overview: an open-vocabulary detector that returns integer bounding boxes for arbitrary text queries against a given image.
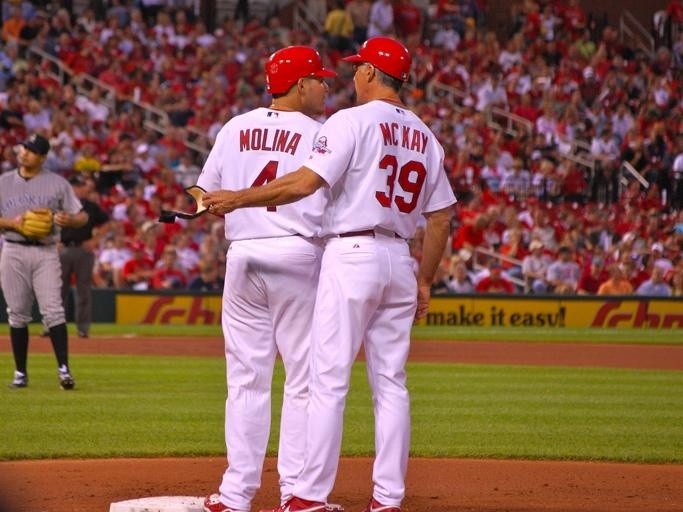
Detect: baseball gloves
[20,208,51,235]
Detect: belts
[5,240,43,246]
[339,230,400,237]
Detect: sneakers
[257,496,326,511]
[78,329,87,337]
[359,497,400,512]
[202,491,235,512]
[43,329,49,336]
[57,363,74,390]
[12,370,27,387]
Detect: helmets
[265,46,337,95]
[341,35,410,83]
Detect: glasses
[351,63,368,76]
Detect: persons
[203,37,458,512]
[42,174,113,340]
[0,1,683,301]
[194,44,344,512]
[0,132,79,393]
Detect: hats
[67,173,85,185]
[22,133,50,155]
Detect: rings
[208,203,215,213]
